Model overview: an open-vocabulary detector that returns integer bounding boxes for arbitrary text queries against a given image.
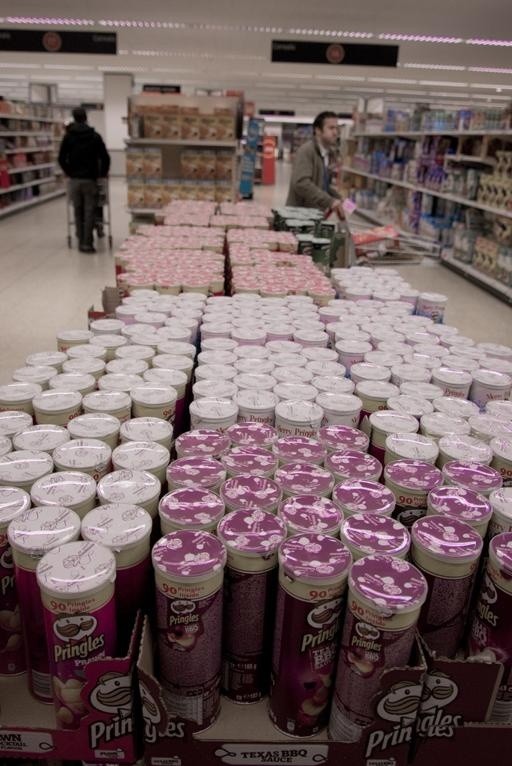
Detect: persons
[87,177,109,240]
[286,111,348,209]
[58,107,113,256]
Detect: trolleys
[335,198,445,271]
[60,175,115,251]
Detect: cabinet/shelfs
[0,94,79,220]
[119,93,243,228]
[330,120,512,304]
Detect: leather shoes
[78,243,97,254]
